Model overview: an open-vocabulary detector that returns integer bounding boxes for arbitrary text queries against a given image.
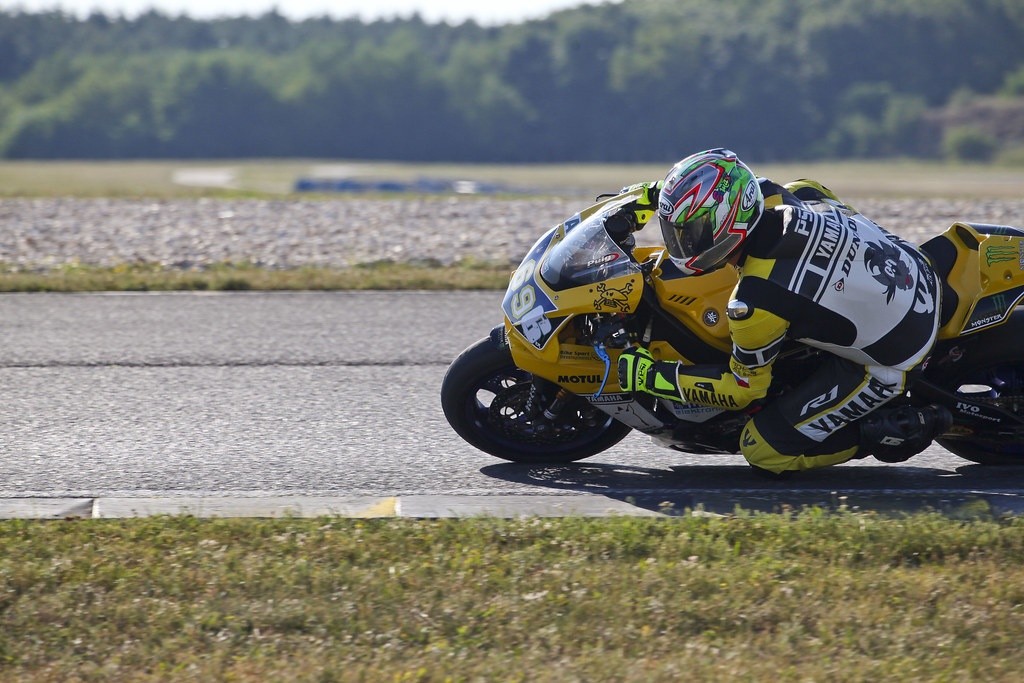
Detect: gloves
[619,180,663,231]
[617,346,686,403]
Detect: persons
[616,149,943,480]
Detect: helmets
[658,148,765,275]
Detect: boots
[853,405,953,463]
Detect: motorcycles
[440,186,1023,466]
